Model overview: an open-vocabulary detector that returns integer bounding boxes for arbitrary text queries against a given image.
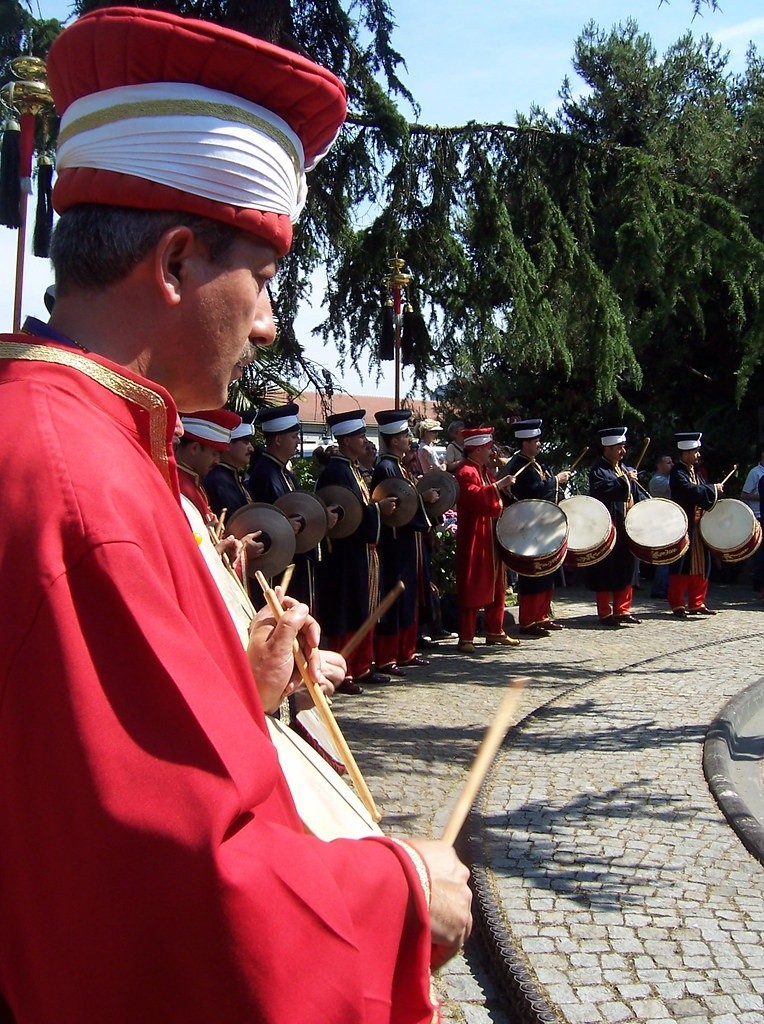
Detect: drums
[294,709,346,778]
[700,498,762,562]
[496,499,569,577]
[558,496,617,566]
[624,498,690,565]
[263,713,389,840]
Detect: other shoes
[334,680,362,694]
[359,672,391,683]
[614,615,641,623]
[458,643,474,653]
[486,635,520,645]
[376,664,405,677]
[673,609,687,618]
[599,615,620,626]
[520,624,551,637]
[398,657,431,666]
[689,606,716,615]
[536,621,564,630]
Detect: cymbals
[222,502,296,579]
[317,484,365,539]
[273,489,329,554]
[370,477,419,527]
[416,470,459,518]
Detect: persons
[170,404,763,711]
[0,5,473,1023]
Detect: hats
[374,410,411,437]
[50,7,345,252]
[419,419,443,431]
[512,419,542,441]
[327,409,367,438]
[181,408,240,451]
[673,432,702,450]
[260,403,301,435]
[598,426,628,447]
[232,410,259,443]
[462,426,495,447]
